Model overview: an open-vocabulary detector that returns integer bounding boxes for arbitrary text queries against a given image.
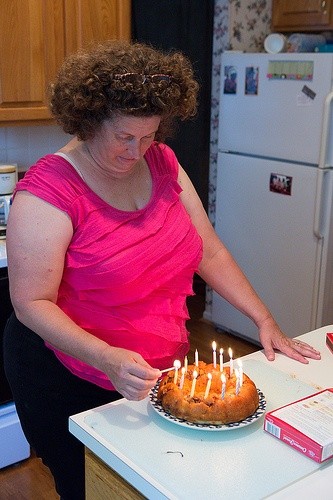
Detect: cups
[286,33,326,53]
[264,33,289,54]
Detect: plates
[147,376,268,431]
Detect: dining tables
[69,325,333,500]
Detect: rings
[296,342,300,346]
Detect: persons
[3,40,322,500]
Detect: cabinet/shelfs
[272,0,333,33]
[0,0,131,127]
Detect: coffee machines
[0,164,18,246]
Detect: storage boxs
[264,388,333,464]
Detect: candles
[173,341,243,399]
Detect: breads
[157,361,259,425]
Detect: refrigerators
[201,51,333,348]
